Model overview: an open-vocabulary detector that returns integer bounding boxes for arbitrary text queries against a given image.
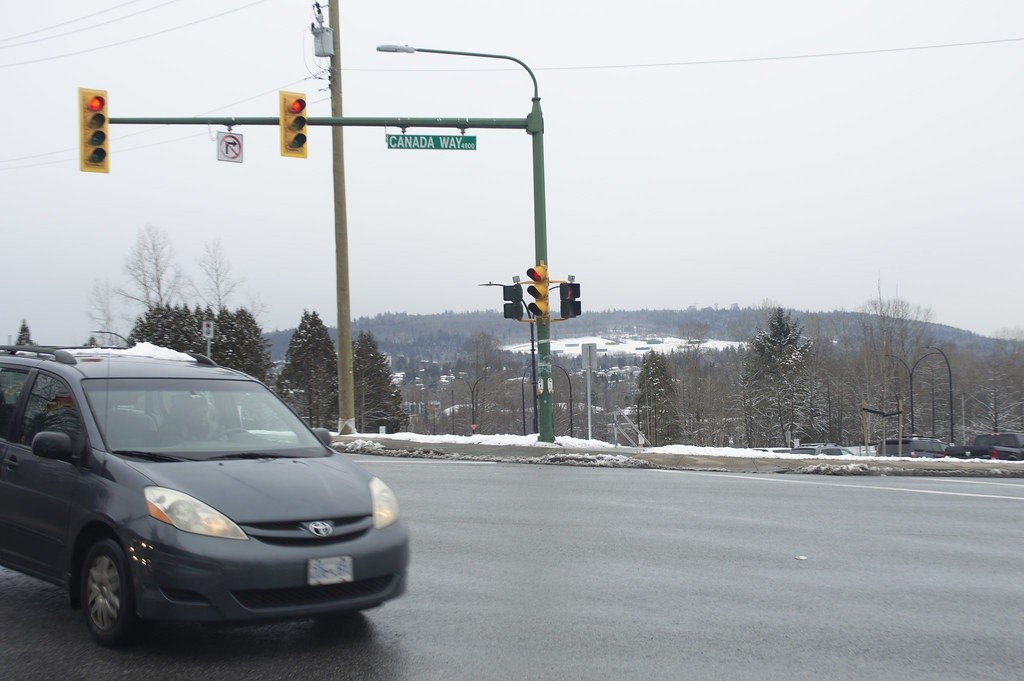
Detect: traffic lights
[560,284,581,318]
[503,281,522,324]
[278,92,308,157]
[526,264,549,320]
[79,87,110,173]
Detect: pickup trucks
[943,432,1024,460]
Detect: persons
[162,395,228,447]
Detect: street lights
[920,344,954,443]
[553,365,575,438]
[882,353,941,437]
[522,364,533,435]
[479,281,559,435]
[458,376,489,434]
[91,330,129,342]
[377,44,555,442]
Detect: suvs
[0,345,410,649]
[753,447,792,453]
[876,435,949,459]
[792,443,855,455]
